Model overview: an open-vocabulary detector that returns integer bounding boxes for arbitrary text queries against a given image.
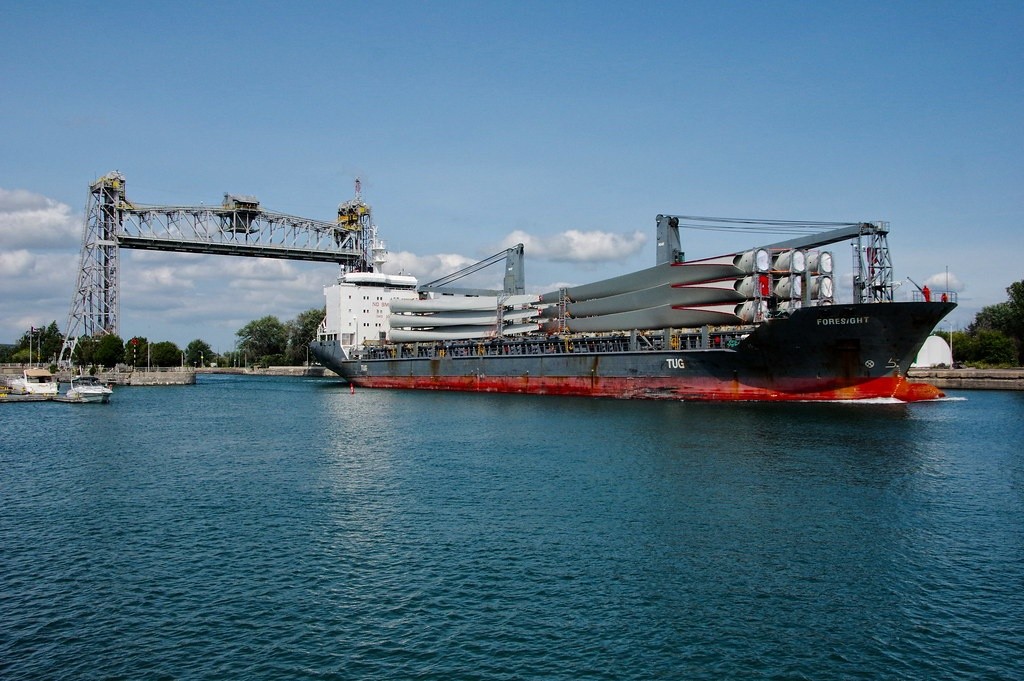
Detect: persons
[362,331,737,360]
[922,285,931,302]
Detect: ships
[311,213,963,406]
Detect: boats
[53,375,114,404]
[0,363,60,401]
